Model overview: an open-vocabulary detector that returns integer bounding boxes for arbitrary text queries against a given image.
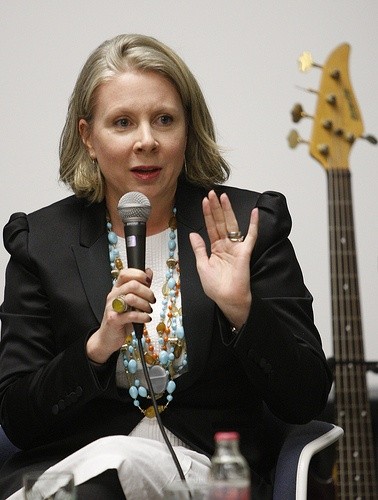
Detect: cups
[22,470,78,500]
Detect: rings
[228,232,245,243]
[112,296,127,314]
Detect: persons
[0,33,332,500]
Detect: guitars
[288,41,377,500]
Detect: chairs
[0,421,343,500]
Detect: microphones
[116,192,151,339]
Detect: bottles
[206,432,251,500]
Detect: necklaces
[105,209,187,419]
